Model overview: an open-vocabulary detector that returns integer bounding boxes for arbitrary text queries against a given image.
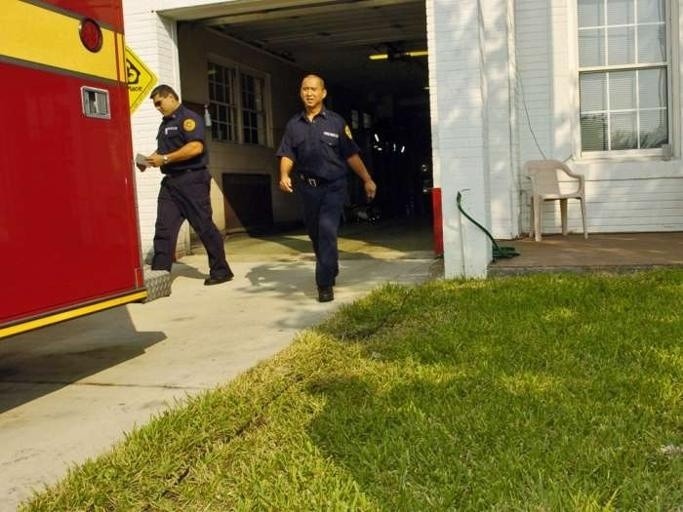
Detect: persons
[274,74,376,302]
[137,85,234,286]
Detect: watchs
[163,154,169,165]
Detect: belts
[300,173,324,187]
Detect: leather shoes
[205,277,232,285]
[319,286,334,302]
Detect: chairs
[523,161,590,242]
